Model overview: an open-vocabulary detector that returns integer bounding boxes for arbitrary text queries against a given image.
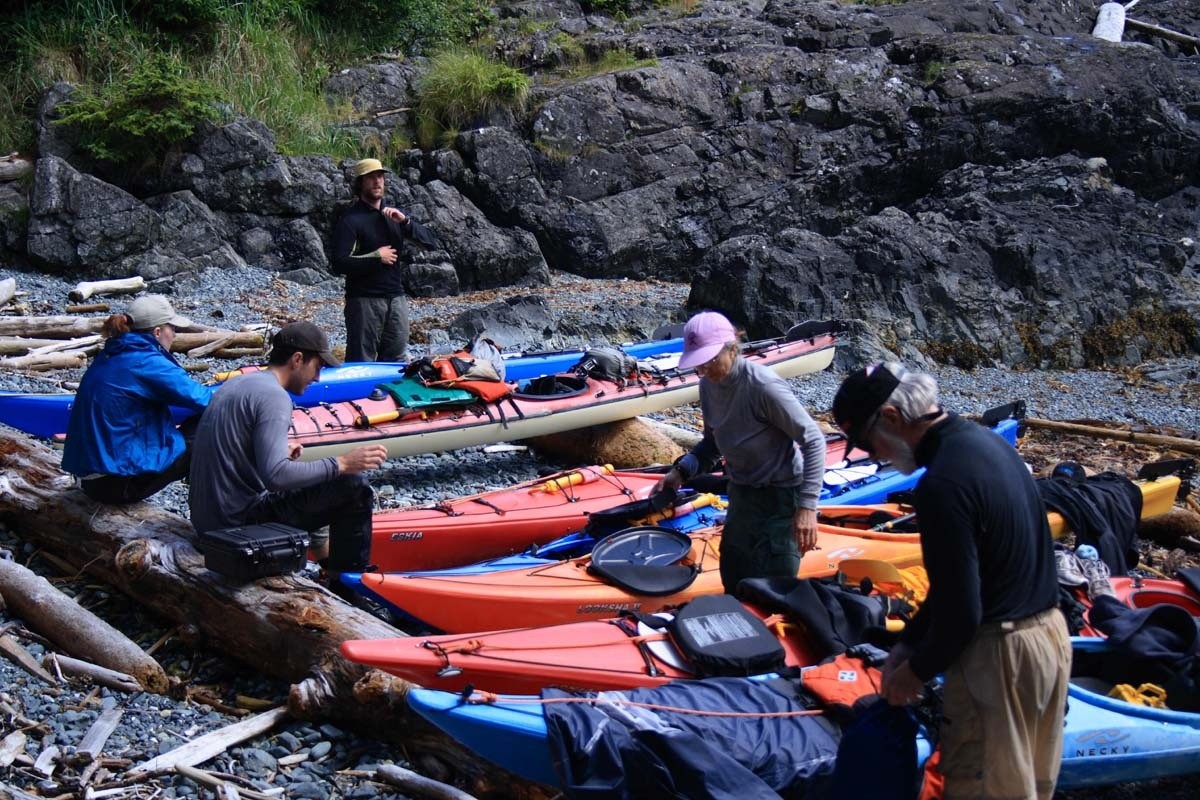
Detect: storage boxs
[200,520,318,580]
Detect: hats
[354,158,391,178]
[273,321,341,368]
[123,295,194,330]
[677,312,736,369]
[831,361,905,462]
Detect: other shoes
[328,578,395,624]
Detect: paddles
[838,559,906,582]
[867,512,918,532]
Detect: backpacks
[566,348,653,398]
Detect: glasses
[702,356,720,368]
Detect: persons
[61,291,225,507]
[651,311,830,608]
[188,323,389,574]
[831,360,1072,799]
[329,158,438,363]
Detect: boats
[289,327,839,472]
[340,572,1200,695]
[404,638,1200,800]
[308,417,1184,636]
[1,336,684,440]
